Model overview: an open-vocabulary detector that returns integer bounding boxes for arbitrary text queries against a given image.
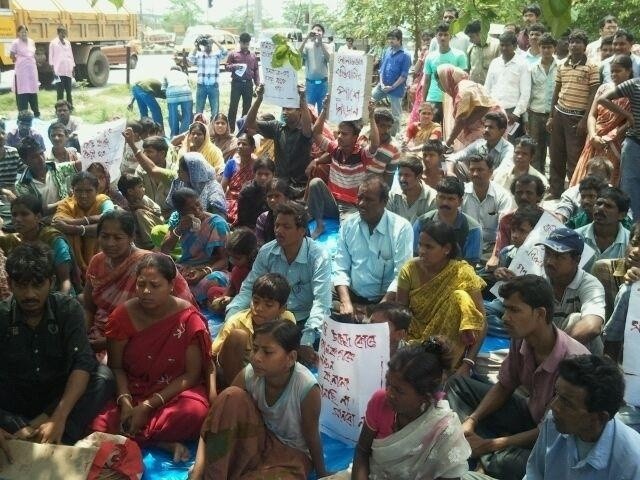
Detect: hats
[535,228,584,254]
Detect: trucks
[0,1,137,88]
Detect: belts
[306,77,327,84]
[237,80,250,83]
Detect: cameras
[311,32,319,37]
[199,36,209,45]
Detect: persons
[0,4,640,480]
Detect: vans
[251,28,304,64]
[174,28,239,70]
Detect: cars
[86,39,142,72]
